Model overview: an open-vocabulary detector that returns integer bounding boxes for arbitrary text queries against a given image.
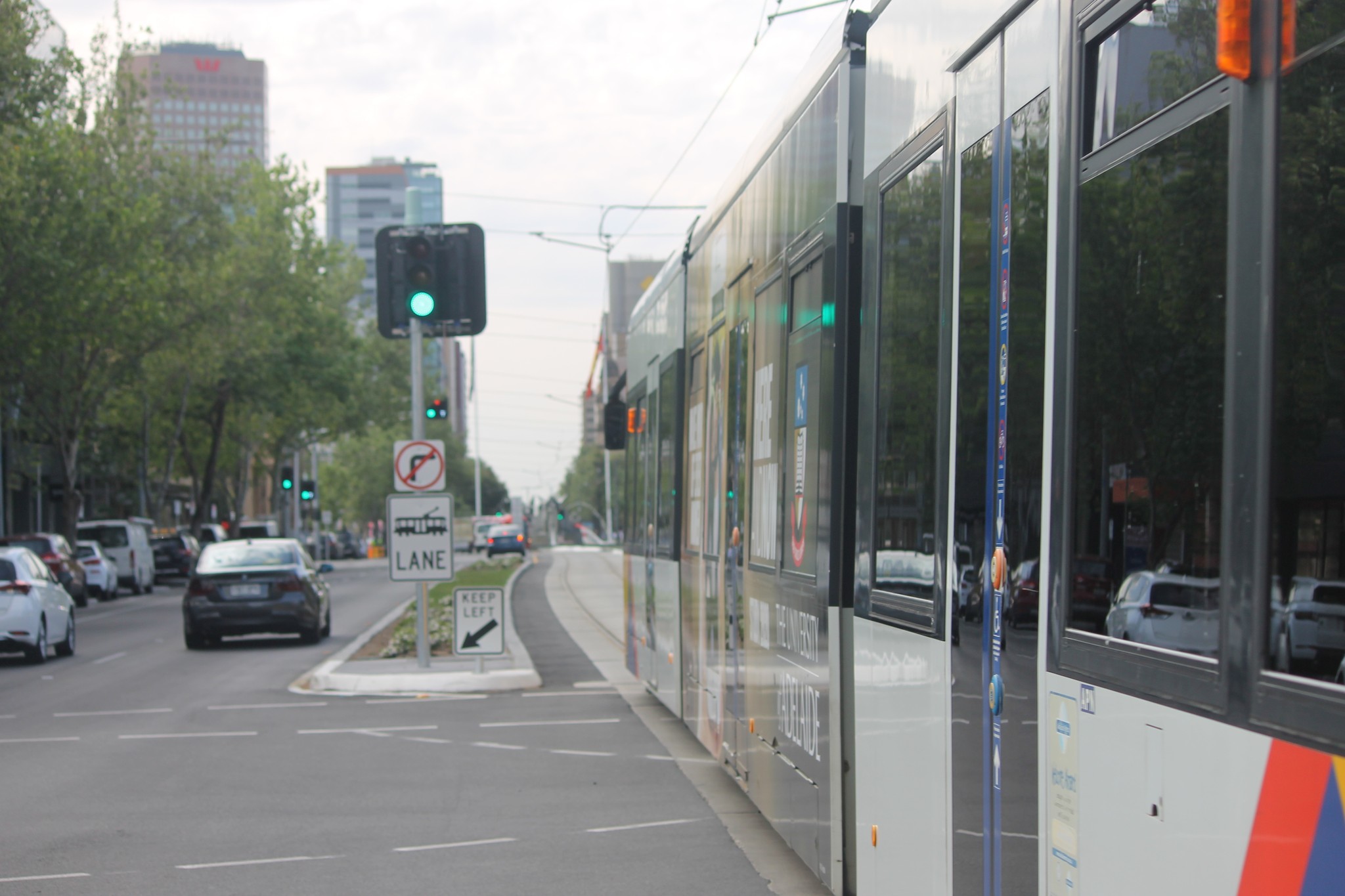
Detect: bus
[601,0,1345,895]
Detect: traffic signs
[452,586,506,657]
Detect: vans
[74,519,158,595]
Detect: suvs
[1105,568,1221,658]
[1267,572,1345,677]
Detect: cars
[306,529,367,561]
[148,534,200,580]
[76,540,119,603]
[0,533,87,607]
[177,524,229,551]
[452,519,528,558]
[232,520,281,540]
[183,536,333,651]
[0,546,78,665]
[856,546,1111,647]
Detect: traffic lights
[425,399,447,420]
[408,234,441,320]
[301,482,314,500]
[281,467,294,491]
